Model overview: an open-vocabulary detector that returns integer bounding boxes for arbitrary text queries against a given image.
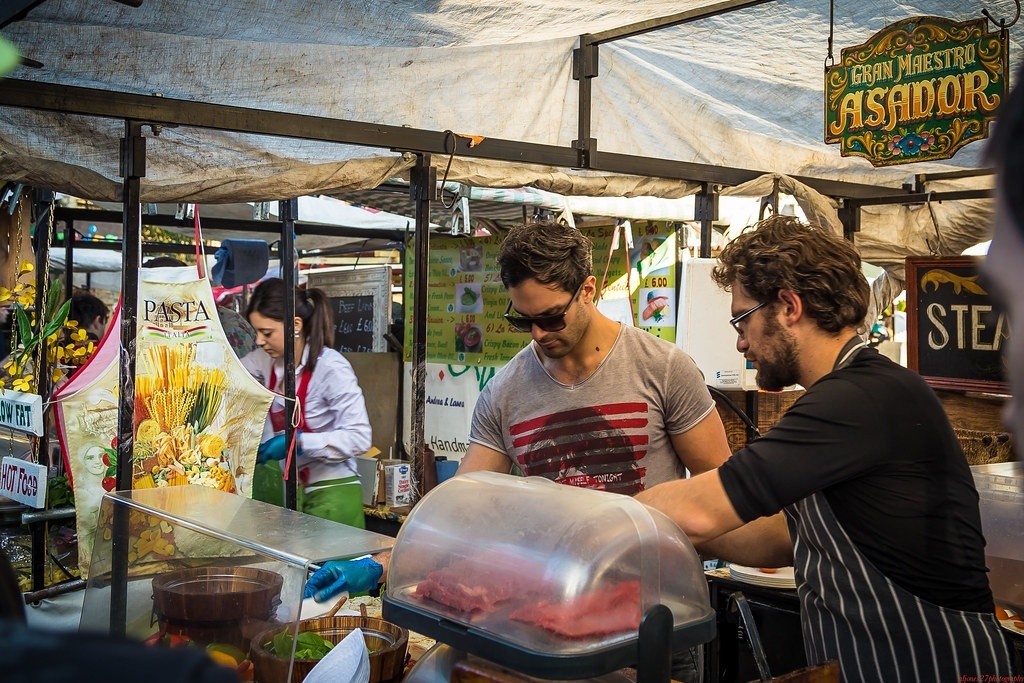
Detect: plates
[999,618,1024,637]
[728,563,797,590]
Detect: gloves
[255,431,304,467]
[303,557,383,604]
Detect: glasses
[729,300,768,334]
[504,283,583,333]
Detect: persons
[973,53,1024,507]
[59,289,107,382]
[614,211,1013,683]
[299,218,734,607]
[229,278,373,532]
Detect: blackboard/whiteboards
[307,265,393,353]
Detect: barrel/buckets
[252,614,408,683]
[149,566,284,653]
